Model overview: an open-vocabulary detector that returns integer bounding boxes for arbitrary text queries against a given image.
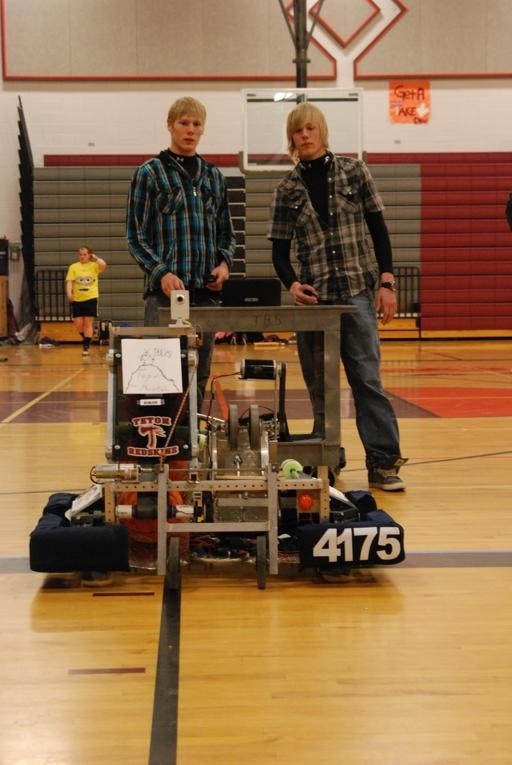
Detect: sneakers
[82,351,89,356]
[368,466,406,491]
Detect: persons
[125,96,239,427]
[264,100,410,493]
[63,245,108,357]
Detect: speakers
[222,277,282,307]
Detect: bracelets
[380,281,397,292]
